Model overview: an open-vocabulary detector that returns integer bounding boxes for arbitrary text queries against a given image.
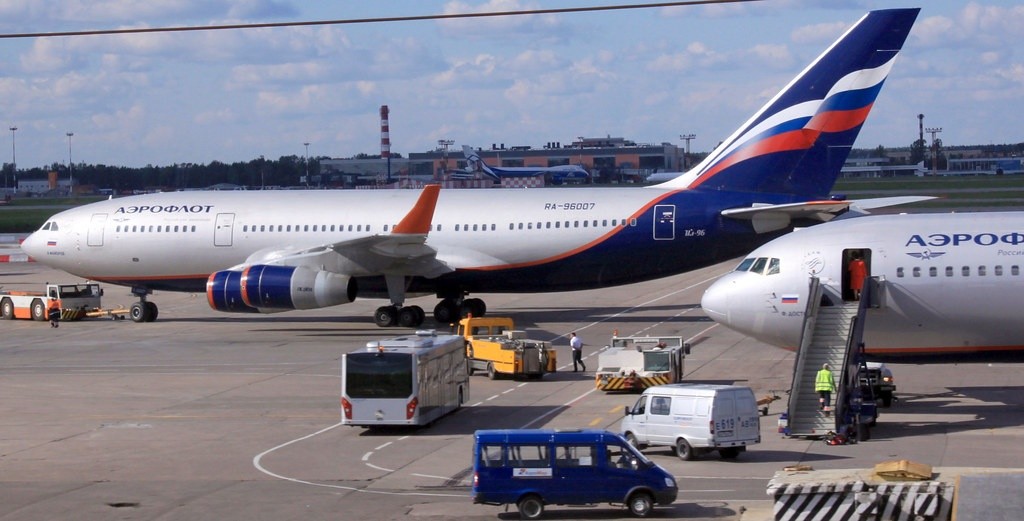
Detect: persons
[815,362,838,417]
[847,251,868,300]
[47,290,60,328]
[570,333,586,372]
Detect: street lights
[10,128,18,193]
[304,142,309,187]
[66,133,74,192]
[577,136,584,165]
[260,155,265,190]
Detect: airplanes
[19,7,941,327]
[443,145,589,185]
[701,211,1024,363]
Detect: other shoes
[572,369,578,372]
[583,366,586,371]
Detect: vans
[620,383,762,461]
[471,430,679,520]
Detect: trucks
[857,362,896,408]
[0,280,104,321]
[450,313,556,381]
[595,329,690,394]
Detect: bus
[341,329,474,425]
